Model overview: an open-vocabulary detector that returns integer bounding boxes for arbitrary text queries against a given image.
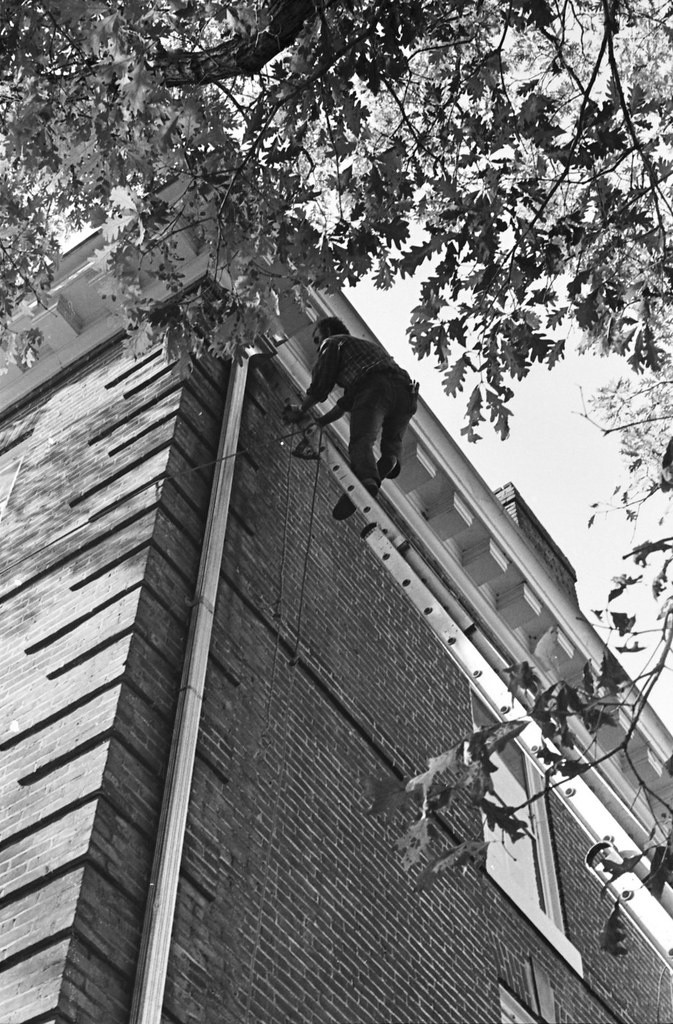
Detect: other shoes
[376,454,396,480]
[333,478,378,520]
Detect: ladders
[290,404,673,978]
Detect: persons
[283,317,420,519]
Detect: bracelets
[298,404,305,413]
